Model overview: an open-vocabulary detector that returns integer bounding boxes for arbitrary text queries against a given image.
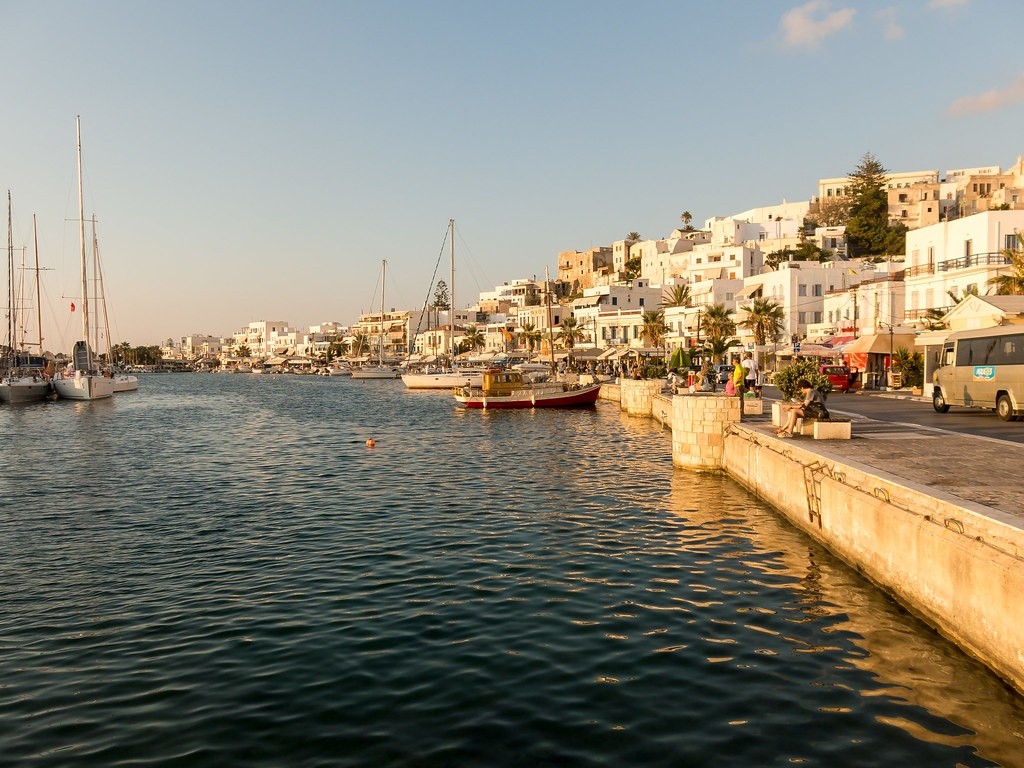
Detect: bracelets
[791,405,793,409]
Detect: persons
[841,361,852,394]
[777,379,829,439]
[564,361,637,378]
[741,351,758,392]
[732,357,745,421]
[755,366,764,398]
[772,405,801,434]
[465,379,471,389]
[721,370,737,397]
[694,371,712,391]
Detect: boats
[400,214,502,389]
[52,113,116,400]
[191,352,404,379]
[0,185,52,405]
[454,261,604,416]
[90,213,139,394]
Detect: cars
[713,365,734,383]
[818,364,852,392]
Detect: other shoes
[774,428,793,438]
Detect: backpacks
[810,402,830,418]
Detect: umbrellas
[666,346,693,369]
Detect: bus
[928,323,1024,422]
